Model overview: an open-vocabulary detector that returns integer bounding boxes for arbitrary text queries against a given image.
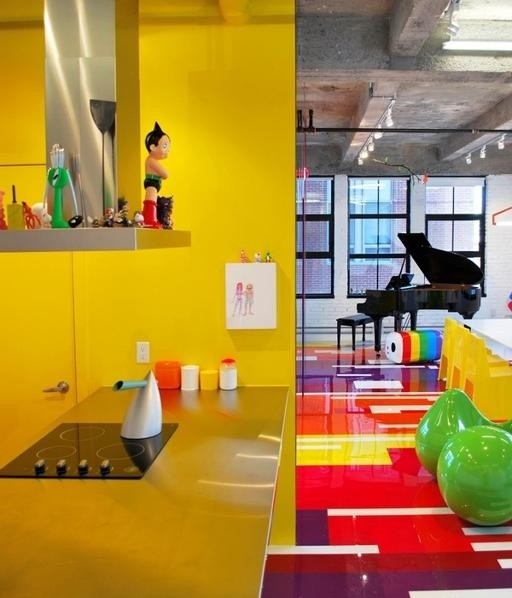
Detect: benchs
[335,314,374,351]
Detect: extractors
[1,0,193,255]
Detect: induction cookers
[0,423,179,477]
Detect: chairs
[435,318,511,423]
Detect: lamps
[358,109,394,169]
[465,134,506,164]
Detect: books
[385,272,414,289]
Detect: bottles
[153,359,238,391]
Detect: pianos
[357,233,485,354]
